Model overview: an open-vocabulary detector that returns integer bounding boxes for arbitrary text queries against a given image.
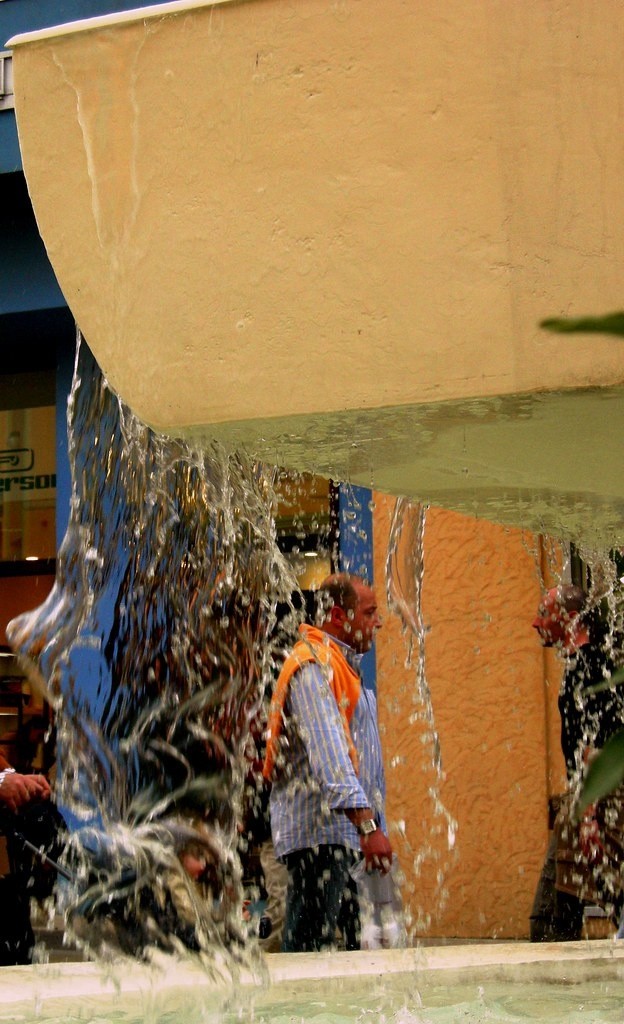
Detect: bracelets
[0,768,15,784]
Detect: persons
[261,573,393,953]
[530,584,624,943]
[0,754,51,966]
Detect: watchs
[357,817,379,834]
